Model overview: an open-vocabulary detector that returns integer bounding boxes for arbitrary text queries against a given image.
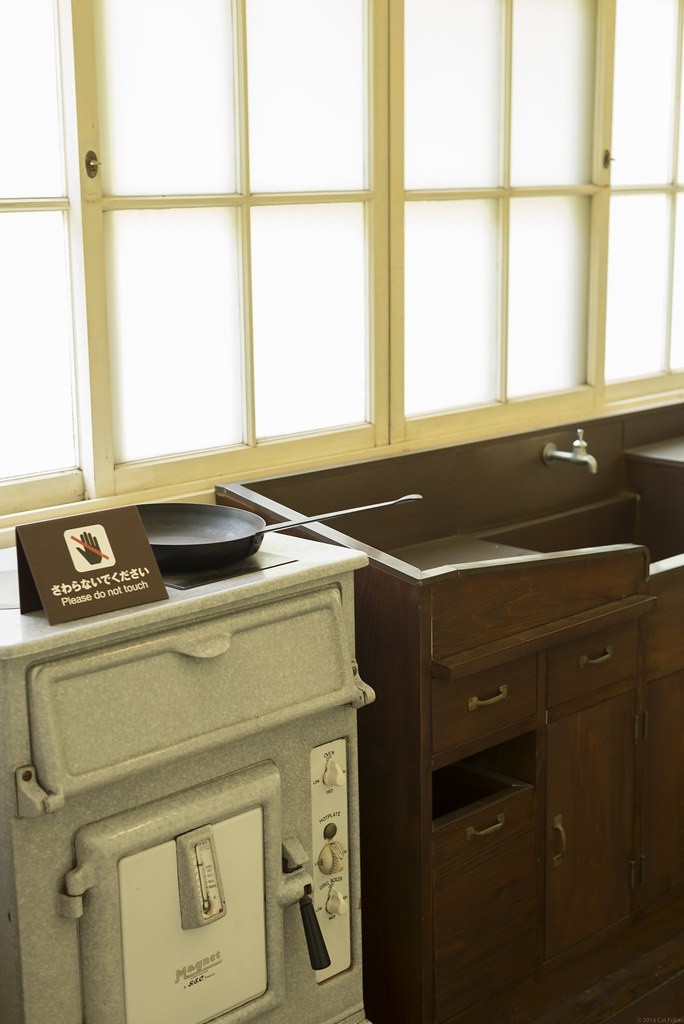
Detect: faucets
[551,428,598,475]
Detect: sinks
[476,486,684,571]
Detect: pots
[133,493,423,571]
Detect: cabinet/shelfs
[359,600,684,1024]
